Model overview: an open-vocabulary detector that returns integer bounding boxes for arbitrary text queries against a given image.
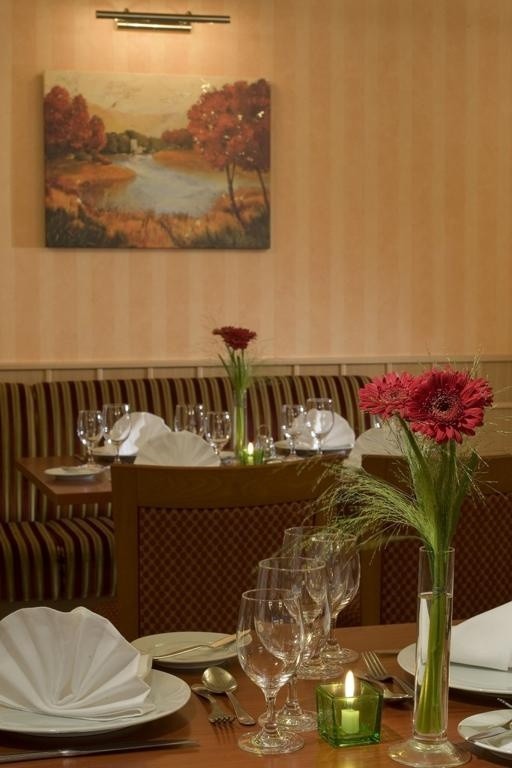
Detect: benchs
[1,374,400,606]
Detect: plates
[130,632,252,669]
[456,710,511,759]
[2,668,192,738]
[397,625,511,702]
[274,440,353,453]
[43,465,103,482]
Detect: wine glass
[234,525,360,757]
[76,398,335,473]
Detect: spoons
[352,670,413,705]
[201,665,258,726]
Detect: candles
[341,708,361,736]
[246,455,256,465]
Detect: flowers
[269,347,512,733]
[204,322,263,445]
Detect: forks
[361,650,414,699]
[190,683,236,729]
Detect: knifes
[149,629,251,662]
[0,737,197,763]
[464,719,511,743]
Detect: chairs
[105,452,351,644]
[355,451,512,630]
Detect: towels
[132,427,225,472]
[0,601,159,726]
[109,408,170,456]
[286,405,353,454]
[341,421,422,481]
[446,595,512,677]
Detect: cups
[315,679,381,748]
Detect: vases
[387,545,472,768]
[221,385,250,454]
